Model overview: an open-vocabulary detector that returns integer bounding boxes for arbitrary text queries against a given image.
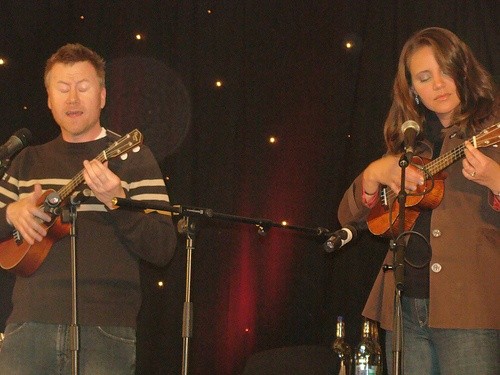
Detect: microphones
[324,225,358,252]
[0,129,32,160]
[402,119,420,156]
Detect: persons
[337,27,500,375]
[0,43,179,375]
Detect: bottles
[333,316,352,375]
[354,317,383,375]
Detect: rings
[472,171,476,176]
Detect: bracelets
[365,190,377,196]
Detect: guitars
[0,128,144,280]
[366,122,500,240]
